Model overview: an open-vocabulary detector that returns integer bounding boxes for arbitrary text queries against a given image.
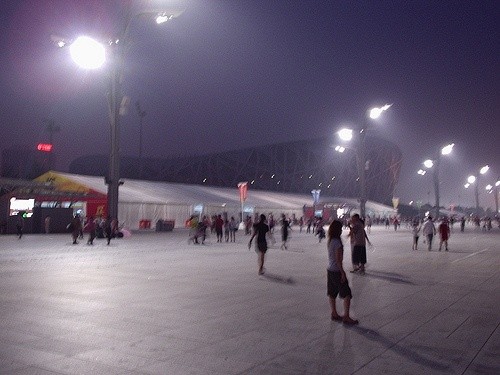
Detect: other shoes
[331,315,342,320]
[343,316,358,325]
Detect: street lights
[424,143,452,222]
[467,165,490,218]
[338,107,383,222]
[485,180,500,213]
[67,5,186,219]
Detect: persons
[358,218,371,273]
[248,215,269,275]
[325,220,360,324]
[45,215,50,233]
[244,214,275,234]
[411,224,421,250]
[328,215,493,234]
[184,214,238,245]
[15,214,24,240]
[68,215,126,247]
[345,214,360,273]
[279,214,292,250]
[421,216,436,251]
[289,213,327,242]
[438,220,450,251]
